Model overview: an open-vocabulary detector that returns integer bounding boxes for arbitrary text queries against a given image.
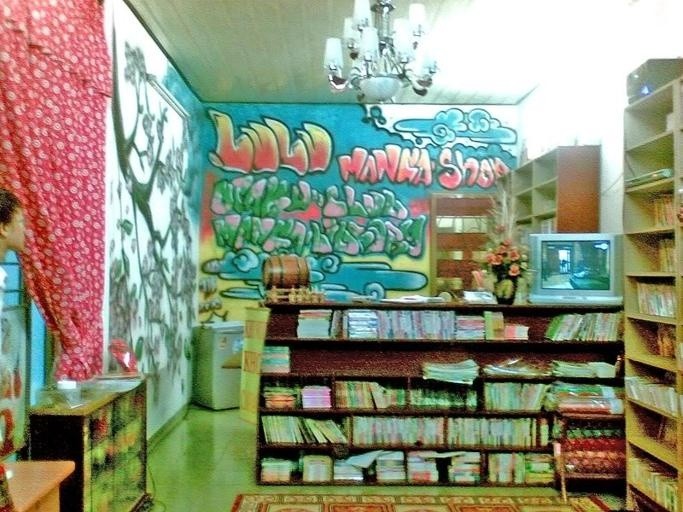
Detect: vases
[494,269,518,305]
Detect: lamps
[325,0,439,100]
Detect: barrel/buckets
[262,254,310,289]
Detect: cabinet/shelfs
[496,145,600,239]
[619,76,683,512]
[255,302,624,484]
[30,372,146,512]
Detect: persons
[0,184,29,322]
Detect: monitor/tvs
[528,233,623,306]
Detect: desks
[0,460,75,512]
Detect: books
[623,166,682,511]
[259,309,625,486]
[351,295,382,304]
[377,298,428,305]
[459,290,499,306]
[401,293,446,303]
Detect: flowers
[485,238,529,276]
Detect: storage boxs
[625,58,683,103]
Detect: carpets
[228,494,614,512]
